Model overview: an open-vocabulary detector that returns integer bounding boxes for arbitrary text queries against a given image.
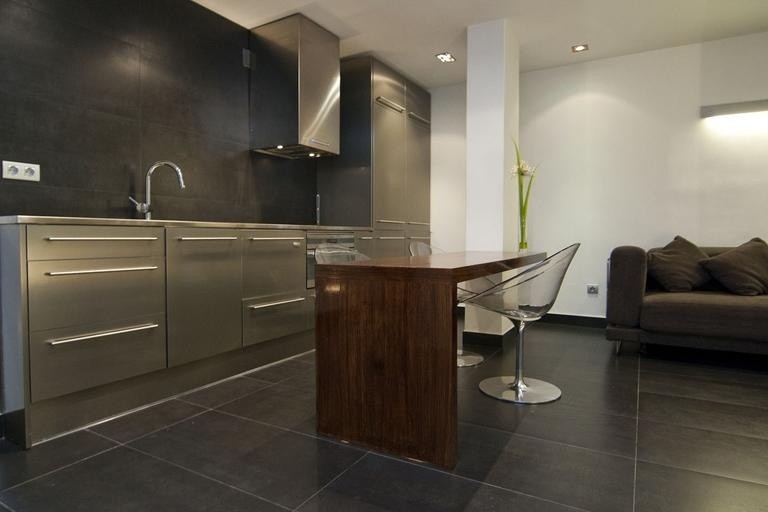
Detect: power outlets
[587,283,599,294]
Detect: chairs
[456,243,581,407]
[407,240,497,365]
[314,242,372,265]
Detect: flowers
[510,138,543,247]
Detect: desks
[315,250,546,475]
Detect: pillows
[646,234,768,295]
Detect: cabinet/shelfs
[307,230,361,333]
[166,232,307,371]
[372,61,434,237]
[28,226,163,406]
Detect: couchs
[606,247,768,355]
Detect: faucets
[127,160,187,220]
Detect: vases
[515,248,537,324]
